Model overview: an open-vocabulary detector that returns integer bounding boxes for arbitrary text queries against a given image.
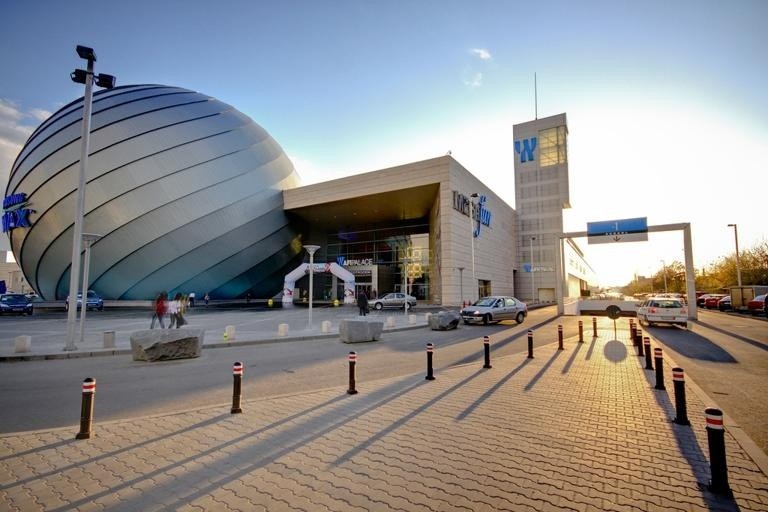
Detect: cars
[368,293,416,311]
[0,292,33,316]
[64,289,103,313]
[633,291,768,326]
[459,295,528,325]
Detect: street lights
[63,43,115,349]
[727,223,742,287]
[77,232,103,343]
[399,256,411,319]
[648,266,653,292]
[457,266,466,304]
[302,243,323,330]
[469,192,480,303]
[530,236,536,304]
[660,260,667,293]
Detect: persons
[357,287,377,316]
[150,290,195,329]
